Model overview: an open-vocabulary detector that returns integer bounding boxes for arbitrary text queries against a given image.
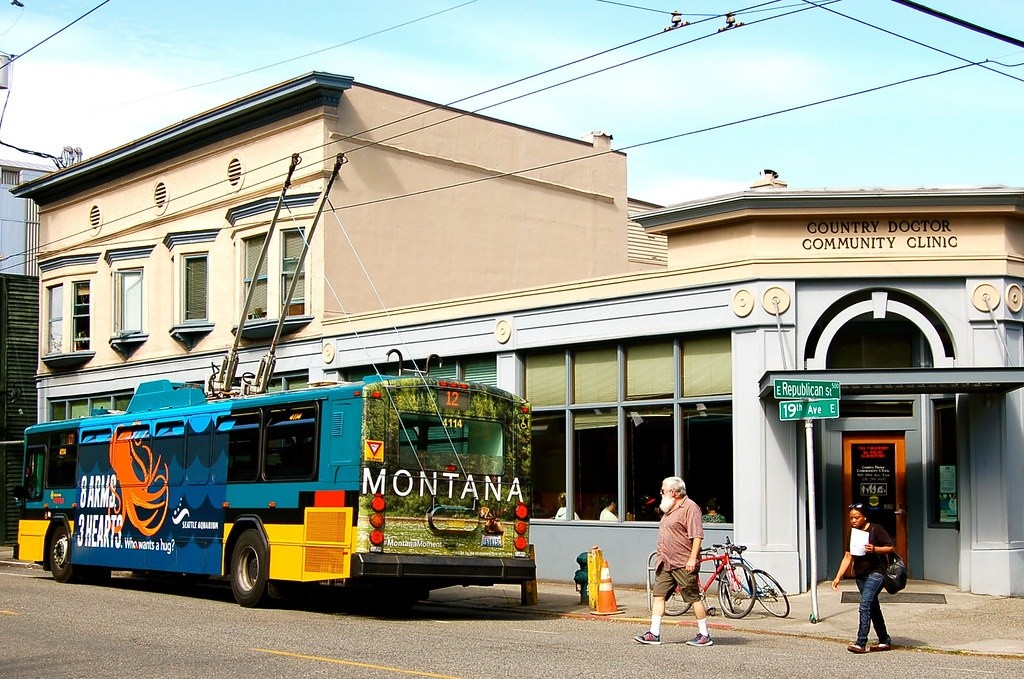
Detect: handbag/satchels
[883,560,907,594]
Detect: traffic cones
[590,560,625,616]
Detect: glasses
[848,503,864,509]
[659,488,665,494]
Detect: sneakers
[634,631,661,644]
[847,645,866,654]
[870,644,892,652]
[686,633,713,647]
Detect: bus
[15,373,537,608]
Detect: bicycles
[663,536,790,619]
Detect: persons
[832,506,894,654]
[599,496,617,520]
[702,499,726,523]
[635,496,661,521]
[555,493,580,520]
[634,477,713,646]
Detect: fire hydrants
[573,551,587,605]
